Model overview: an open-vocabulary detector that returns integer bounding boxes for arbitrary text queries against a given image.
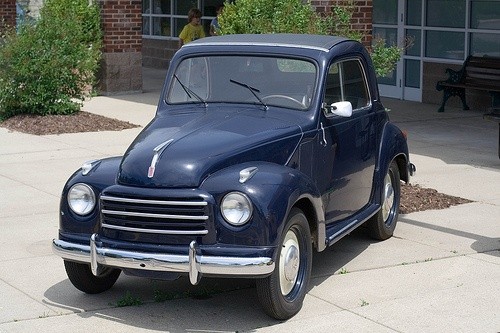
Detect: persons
[210,5,224,36]
[178,8,206,86]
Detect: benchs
[436,55,500,112]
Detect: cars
[52,34,416,320]
